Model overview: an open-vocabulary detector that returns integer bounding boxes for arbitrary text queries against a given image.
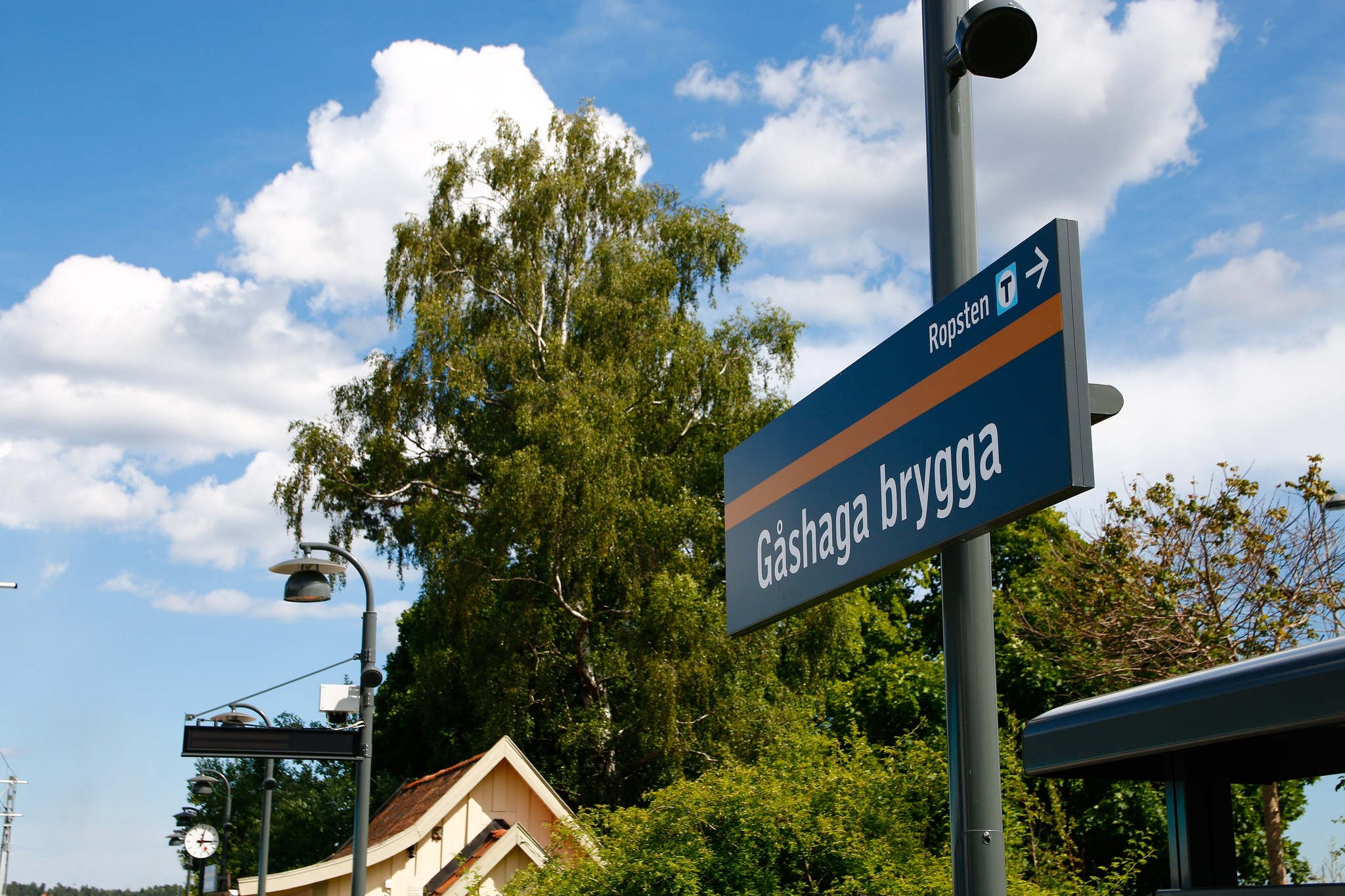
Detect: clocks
[183,822,221,861]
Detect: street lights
[186,769,233,896]
[267,542,382,896]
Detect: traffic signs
[724,220,1095,640]
